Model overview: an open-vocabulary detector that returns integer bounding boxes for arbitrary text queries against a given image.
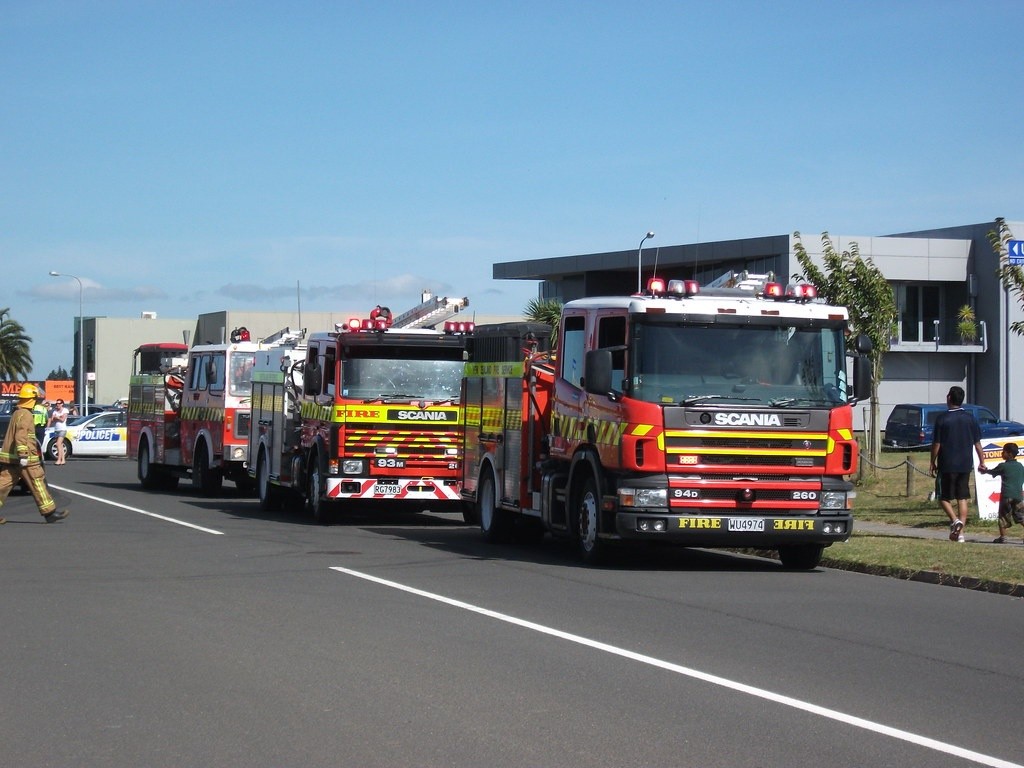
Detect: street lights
[636,232,655,293]
[49,271,84,416]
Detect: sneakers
[949,519,963,541]
[958,536,965,542]
[993,536,1007,543]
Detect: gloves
[19,458,28,466]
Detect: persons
[930,386,986,542]
[45,399,79,465]
[980,443,1024,543]
[0,383,68,523]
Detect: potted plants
[957,319,980,345]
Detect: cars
[0,399,127,458]
[881,403,1023,446]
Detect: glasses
[56,403,62,405]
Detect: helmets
[19,383,39,398]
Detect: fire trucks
[126,270,860,572]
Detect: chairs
[715,340,747,379]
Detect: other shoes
[46,509,69,522]
[0,517,6,523]
[55,460,66,465]
